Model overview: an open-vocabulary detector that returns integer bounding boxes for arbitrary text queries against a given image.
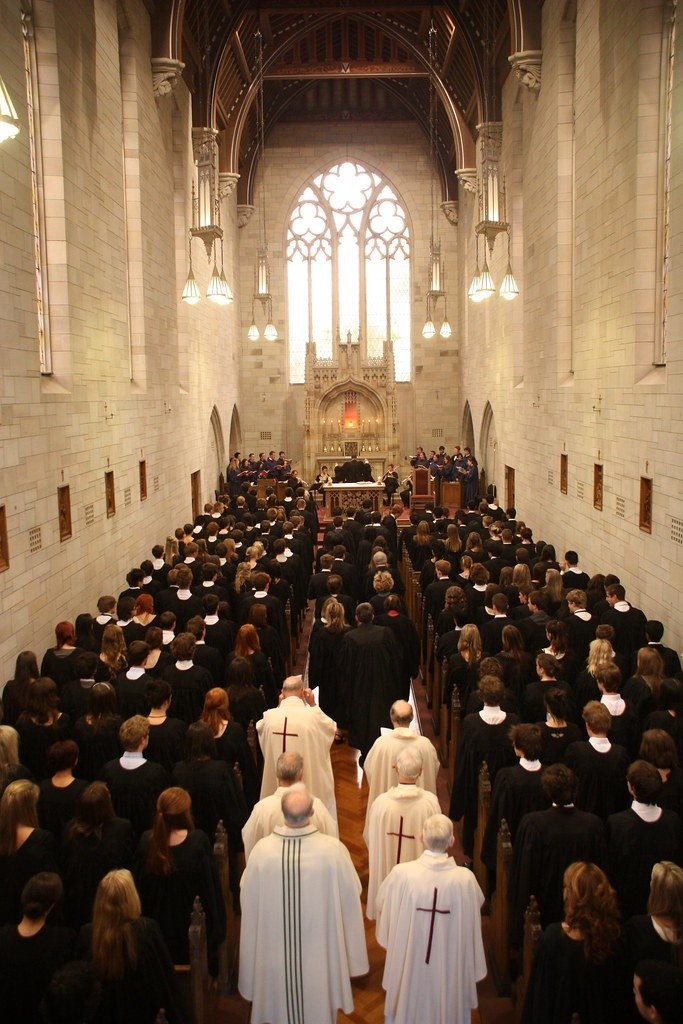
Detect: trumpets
[404,455,417,461]
[296,477,309,487]
[399,475,411,486]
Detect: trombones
[381,465,399,481]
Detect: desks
[322,481,386,521]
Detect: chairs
[409,468,436,514]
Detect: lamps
[245,28,278,341]
[421,18,452,339]
[181,0,235,306]
[0,75,22,144]
[466,0,520,303]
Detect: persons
[0,446,683,1024]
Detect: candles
[322,419,379,453]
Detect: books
[456,466,464,472]
[284,459,292,467]
[237,470,248,477]
[277,465,283,469]
[408,455,417,459]
[261,469,270,476]
